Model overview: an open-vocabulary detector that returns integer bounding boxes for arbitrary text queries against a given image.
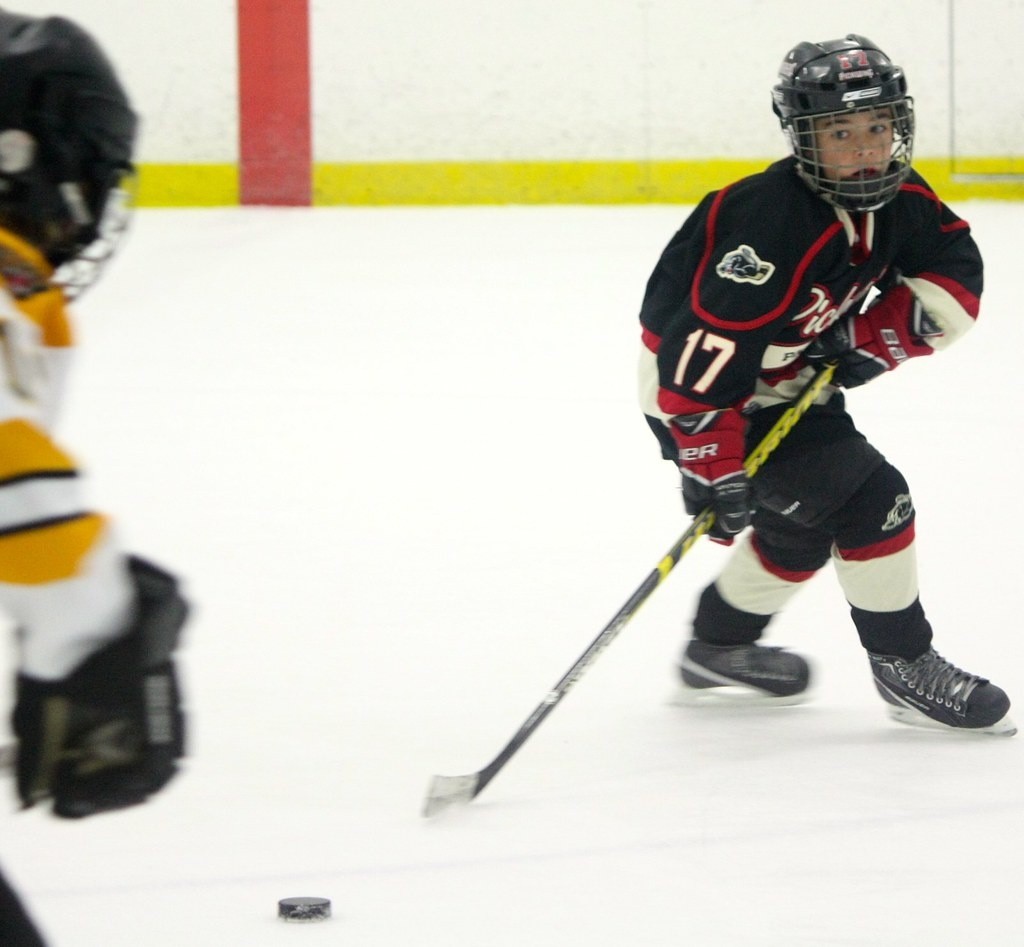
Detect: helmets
[771,34,916,213]
[0,11,138,265]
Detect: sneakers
[681,636,809,706]
[868,645,1017,736]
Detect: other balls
[277,896,330,921]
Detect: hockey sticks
[421,361,843,815]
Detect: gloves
[805,285,945,389]
[666,408,773,546]
[12,557,189,818]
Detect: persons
[0,10,197,946]
[637,35,1017,738]
